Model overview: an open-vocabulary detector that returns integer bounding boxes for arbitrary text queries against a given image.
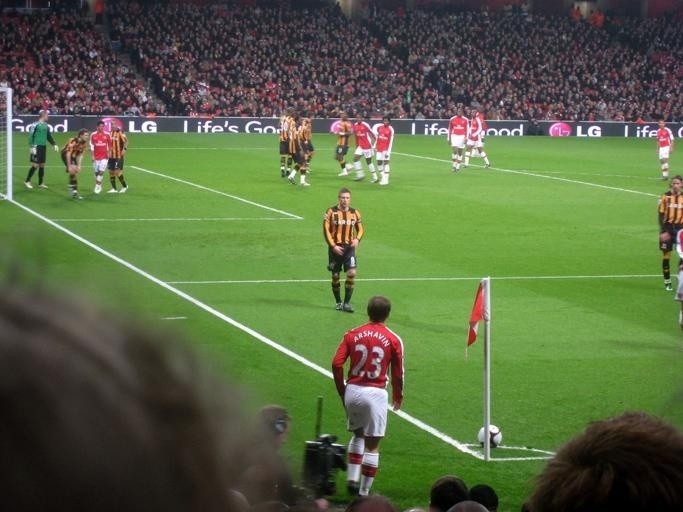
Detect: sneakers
[107,188,118,193]
[664,279,673,291]
[463,165,470,168]
[119,185,128,193]
[337,163,366,181]
[281,167,286,177]
[286,168,290,176]
[484,164,491,169]
[94,184,101,193]
[305,167,312,174]
[370,179,378,183]
[38,183,48,189]
[343,303,354,312]
[24,181,33,188]
[347,481,359,495]
[451,167,460,173]
[336,303,342,310]
[288,177,297,185]
[300,182,311,186]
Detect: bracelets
[122,148,127,151]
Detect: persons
[323,187,364,313]
[0,2,683,124]
[59,128,90,200]
[326,111,353,177]
[470,107,487,158]
[372,117,393,185]
[105,119,128,195]
[288,475,500,512]
[523,410,682,511]
[331,296,405,499]
[0,281,293,511]
[462,109,490,170]
[348,114,378,183]
[88,121,112,195]
[23,109,58,190]
[673,226,683,328]
[657,173,682,290]
[275,108,314,189]
[655,119,673,181]
[445,107,469,174]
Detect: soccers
[478,425,502,448]
